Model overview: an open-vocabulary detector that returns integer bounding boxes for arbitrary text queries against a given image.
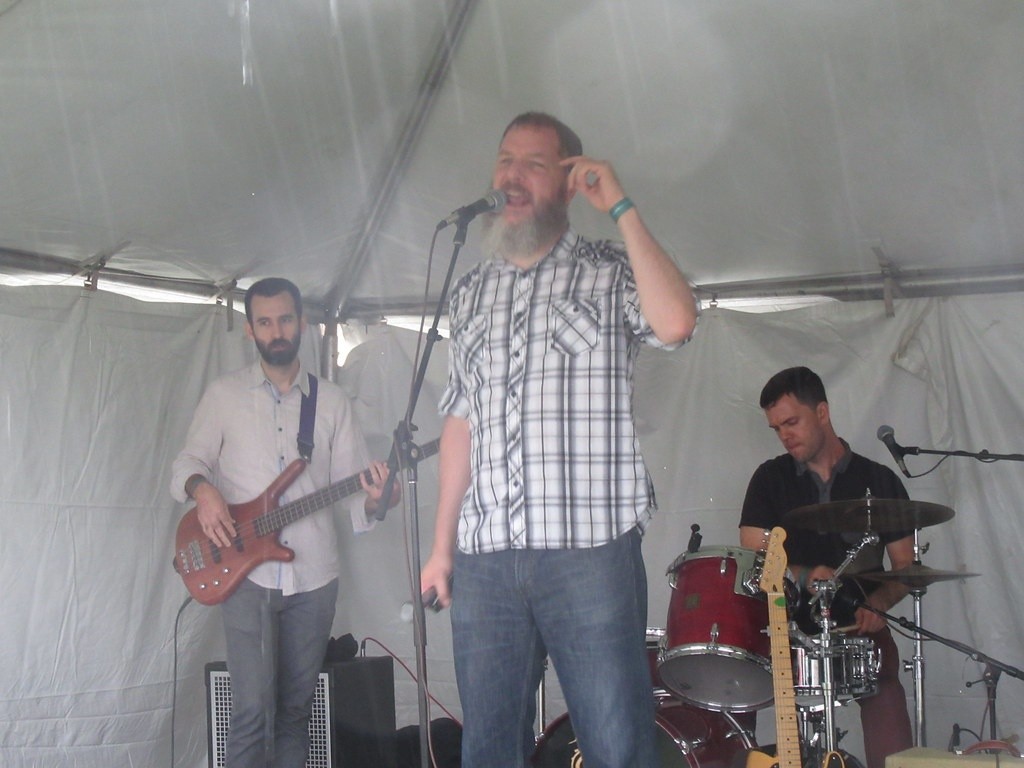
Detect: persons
[733,365,924,768]
[166,276,403,767]
[414,110,699,768]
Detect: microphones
[436,189,506,231]
[876,425,912,479]
[401,586,437,622]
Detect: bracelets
[609,198,636,224]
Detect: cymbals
[784,498,958,540]
[853,555,977,590]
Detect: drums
[533,631,757,768]
[661,546,799,707]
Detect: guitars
[175,427,446,605]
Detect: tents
[0,0,1024,767]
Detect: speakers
[204,656,399,768]
